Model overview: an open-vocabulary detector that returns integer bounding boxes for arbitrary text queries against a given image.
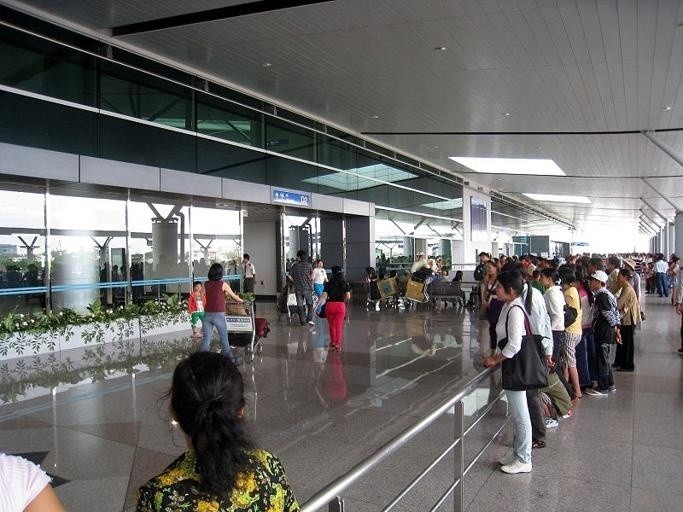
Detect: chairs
[350,281,464,310]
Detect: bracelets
[315,307,317,309]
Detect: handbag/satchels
[564,303,578,329]
[499,306,548,389]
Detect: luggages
[255,318,271,339]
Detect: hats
[591,270,609,283]
[623,259,636,270]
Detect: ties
[245,265,246,276]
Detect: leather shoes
[612,364,634,372]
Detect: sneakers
[193,333,203,337]
[308,321,315,326]
[497,417,558,474]
[331,343,342,351]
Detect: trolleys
[222,300,267,361]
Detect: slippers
[586,386,616,396]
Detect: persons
[244,254,256,292]
[474,251,640,474]
[286,249,350,352]
[366,253,467,312]
[632,253,682,354]
[135,351,300,512]
[189,281,207,336]
[199,263,244,352]
[0,258,236,311]
[0,452,64,512]
